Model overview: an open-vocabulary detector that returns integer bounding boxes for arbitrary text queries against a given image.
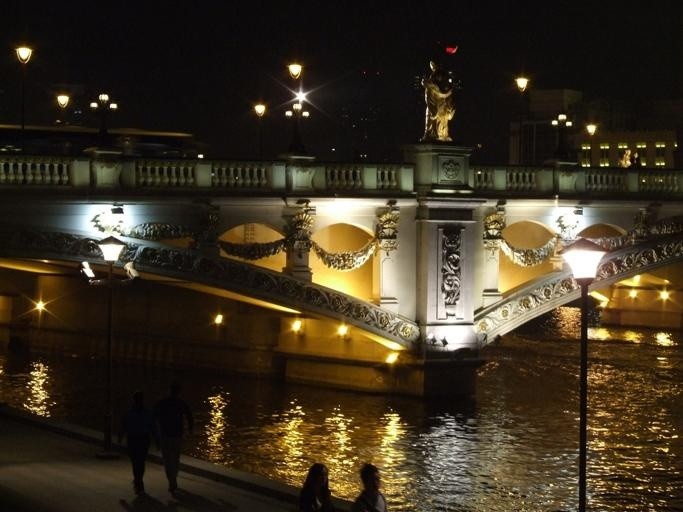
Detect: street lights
[285,103,310,156]
[288,63,303,156]
[552,114,573,162]
[56,95,69,156]
[90,94,118,147]
[556,238,609,512]
[586,124,597,167]
[14,46,32,156]
[514,77,531,163]
[80,237,141,461]
[254,103,266,161]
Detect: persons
[352,464,388,512]
[152,385,194,492]
[117,391,163,495]
[298,463,334,512]
